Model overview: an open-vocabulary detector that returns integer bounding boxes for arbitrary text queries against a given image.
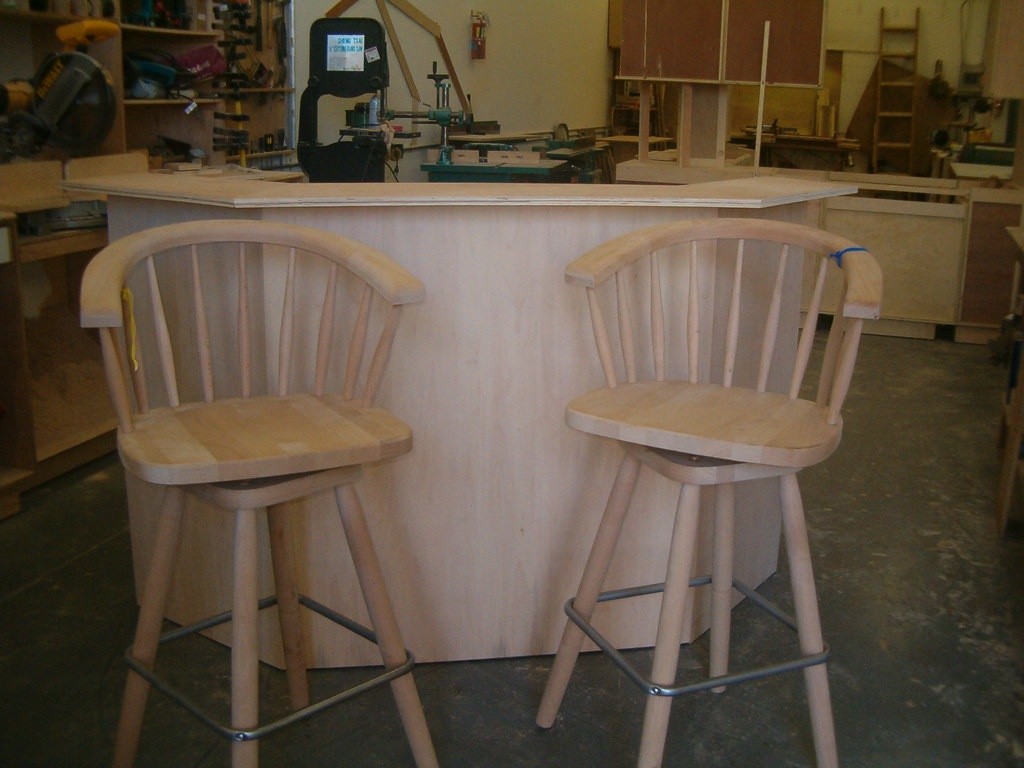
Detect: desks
[730,134,860,170]
[598,135,672,163]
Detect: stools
[80,221,437,768]
[537,217,884,768]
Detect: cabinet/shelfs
[0,0,293,523]
[981,0,1024,98]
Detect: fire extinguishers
[471,9,490,60]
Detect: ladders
[871,6,920,201]
